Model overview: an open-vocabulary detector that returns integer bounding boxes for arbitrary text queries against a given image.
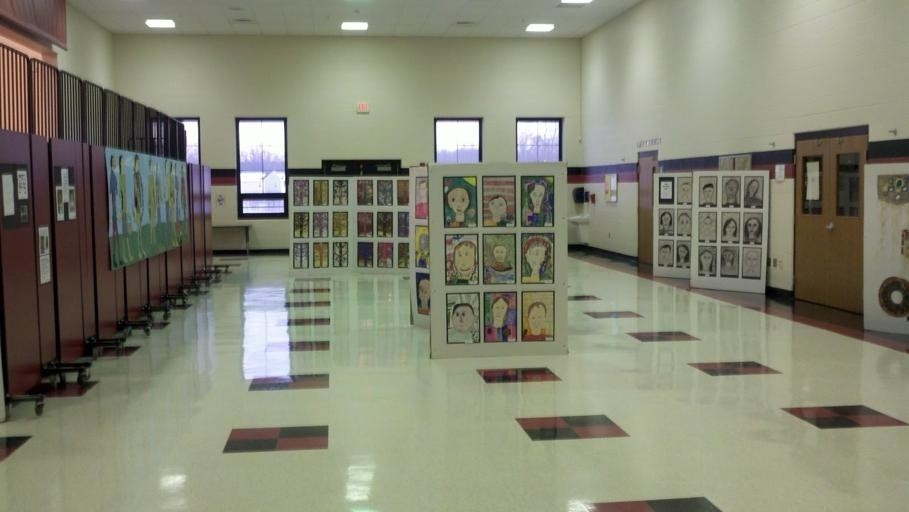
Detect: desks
[212,225,249,257]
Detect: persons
[440,174,558,346]
[656,174,767,281]
[413,175,430,318]
[106,150,192,268]
